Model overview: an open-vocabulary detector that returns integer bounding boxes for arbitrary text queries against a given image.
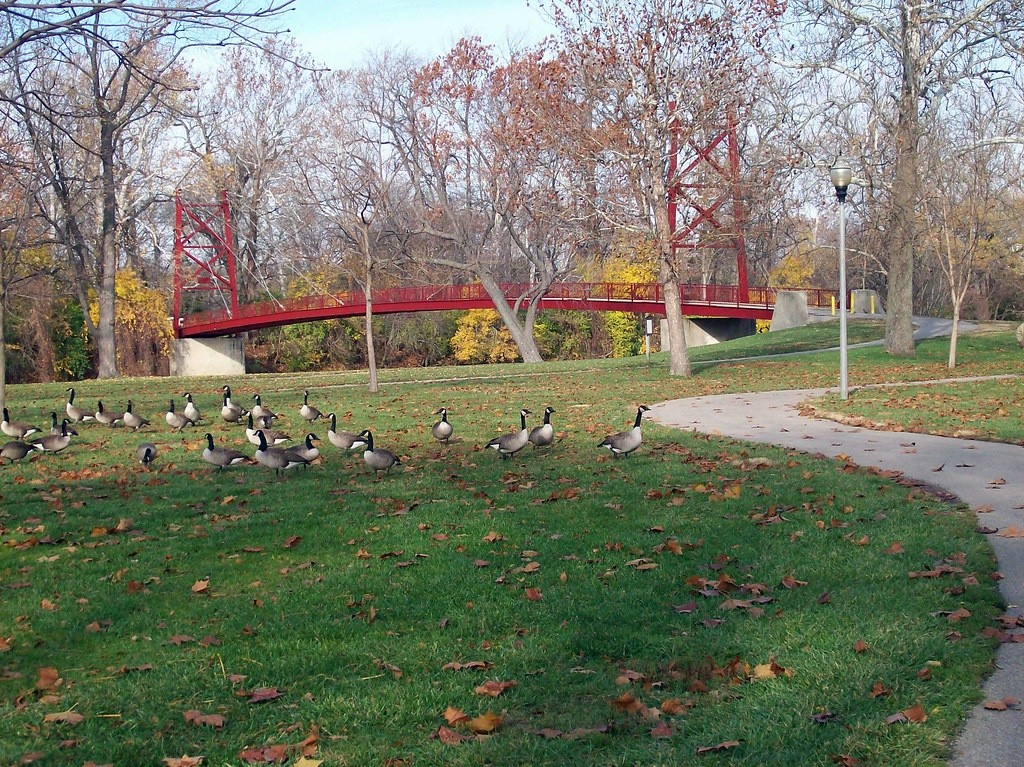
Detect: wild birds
[253,430,310,478]
[47,411,79,438]
[0,439,38,466]
[300,391,325,425]
[136,442,158,468]
[95,400,124,429]
[528,407,556,452]
[241,411,293,448]
[250,394,279,428]
[66,387,203,436]
[598,405,651,458]
[356,430,400,478]
[201,433,252,475]
[28,418,73,455]
[325,413,369,454]
[288,432,320,470]
[220,385,250,427]
[431,407,453,445]
[0,407,43,442]
[485,409,532,461]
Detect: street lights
[829,147,852,402]
